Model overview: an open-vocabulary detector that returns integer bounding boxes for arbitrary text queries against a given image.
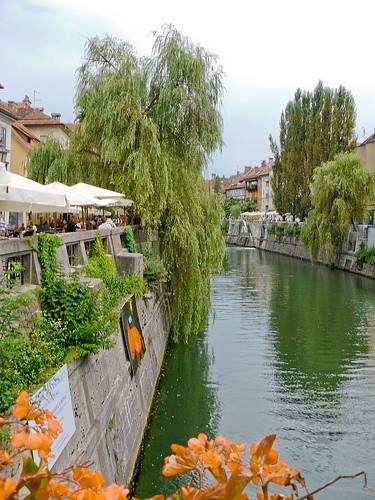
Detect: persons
[5,217,124,239]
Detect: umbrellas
[44,181,134,227]
[0,161,69,215]
